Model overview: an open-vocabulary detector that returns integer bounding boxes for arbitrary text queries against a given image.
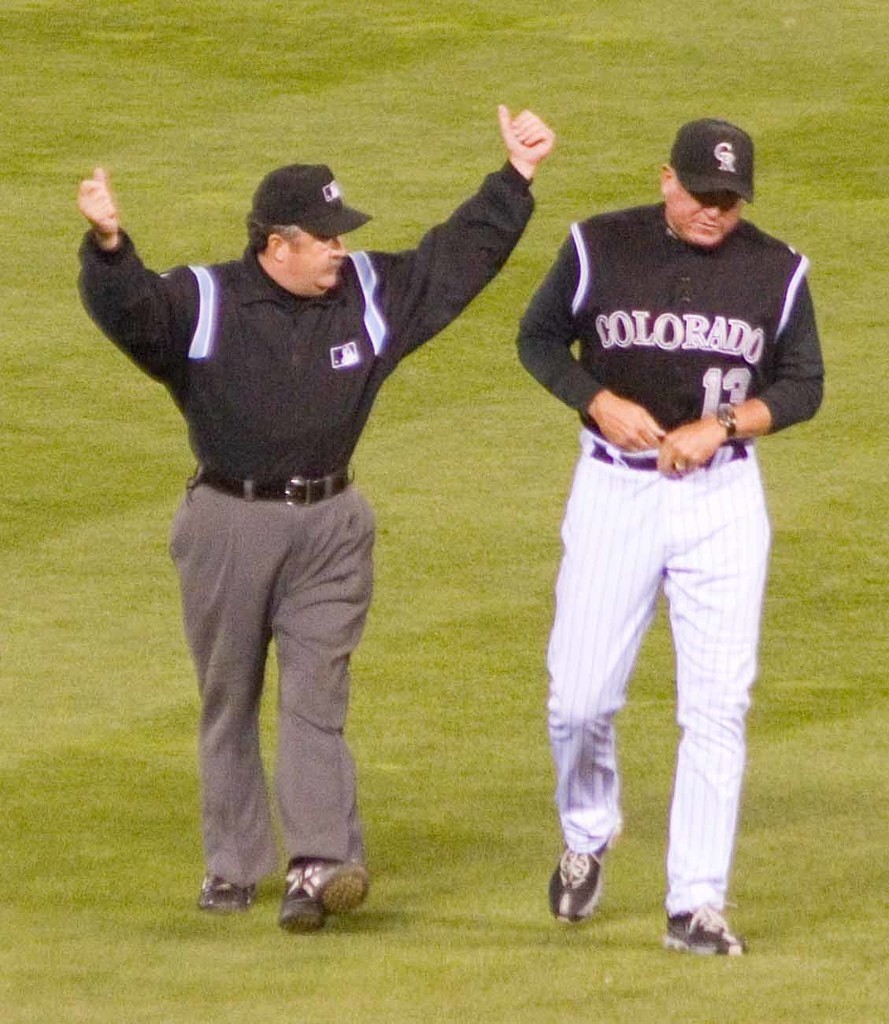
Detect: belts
[202,466,349,506]
[590,438,748,472]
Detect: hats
[252,162,374,237]
[670,118,754,203]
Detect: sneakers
[664,907,744,956]
[548,822,623,924]
[198,868,255,916]
[276,859,369,935]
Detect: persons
[514,120,825,955]
[79,106,552,931]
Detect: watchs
[716,402,740,441]
